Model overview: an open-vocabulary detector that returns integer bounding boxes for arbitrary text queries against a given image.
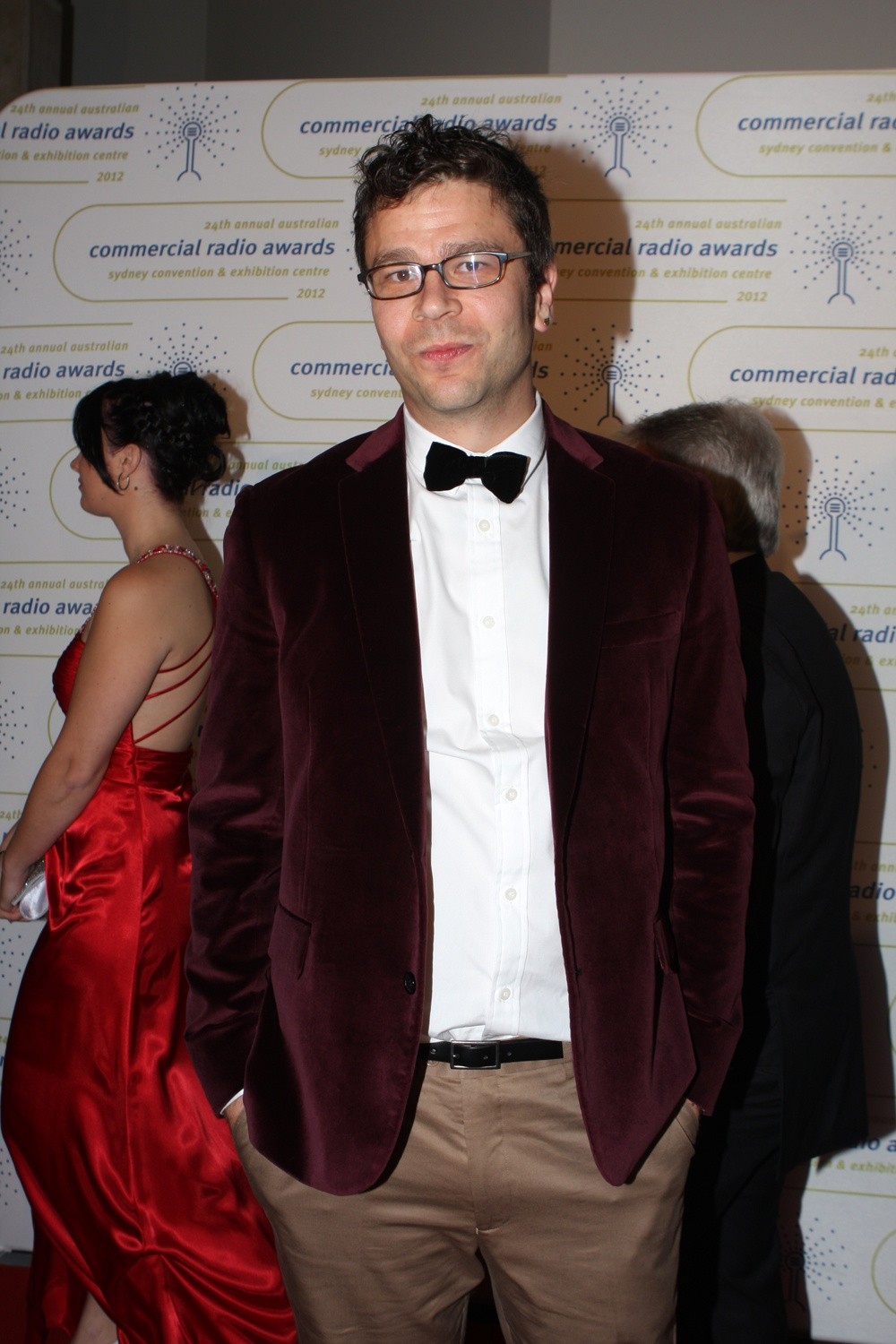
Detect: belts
[425,1038,564,1070]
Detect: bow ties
[424,443,530,504]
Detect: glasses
[358,250,535,301]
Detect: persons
[0,370,298,1344]
[186,114,755,1343]
[619,401,869,1344]
